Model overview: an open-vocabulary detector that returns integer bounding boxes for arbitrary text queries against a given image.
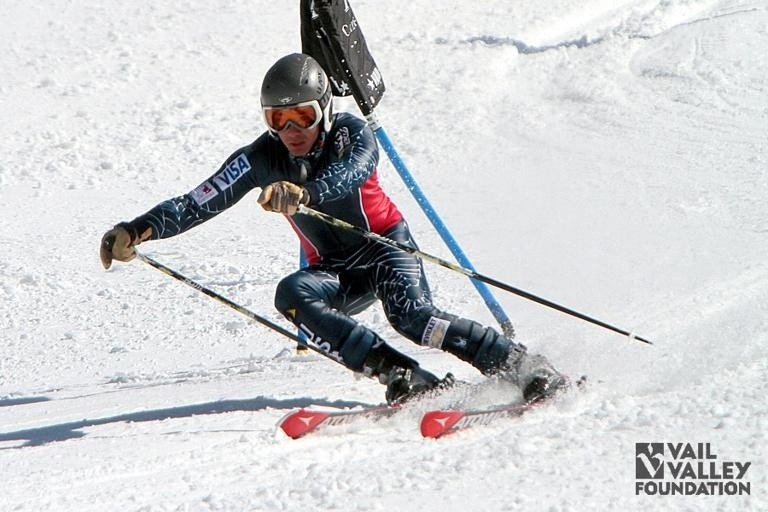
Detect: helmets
[260,54,333,110]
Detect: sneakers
[380,365,440,408]
[516,355,563,404]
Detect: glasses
[262,100,324,133]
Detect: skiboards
[278,374,595,441]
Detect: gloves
[100,219,152,271]
[256,180,310,217]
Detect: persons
[100,52,569,404]
